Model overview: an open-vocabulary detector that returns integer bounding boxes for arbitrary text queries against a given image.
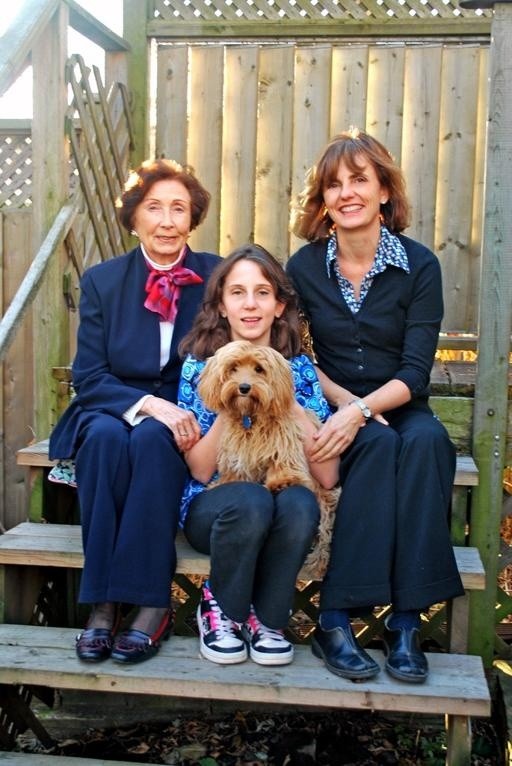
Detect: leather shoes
[310,613,380,679]
[75,602,122,663]
[380,611,429,684]
[110,603,177,665]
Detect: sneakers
[240,597,295,666]
[195,578,250,665]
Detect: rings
[179,433,188,436]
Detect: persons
[177,244,339,668]
[49,161,232,664]
[287,131,457,681]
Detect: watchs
[348,399,372,423]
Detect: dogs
[201,337,343,581]
